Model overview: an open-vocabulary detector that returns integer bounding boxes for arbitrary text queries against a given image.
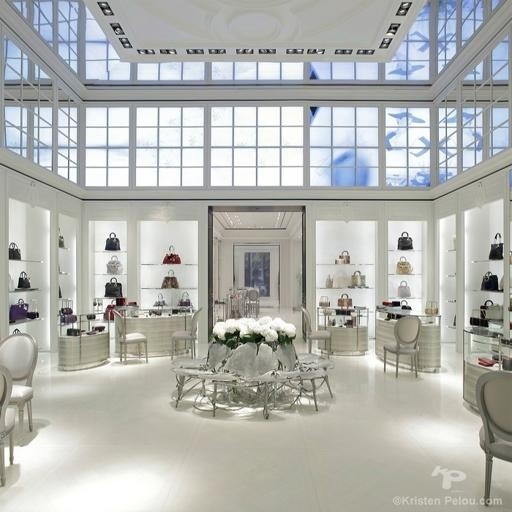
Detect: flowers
[213,316,297,351]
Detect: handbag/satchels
[318,231,512,371]
[8,226,192,336]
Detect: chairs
[215,287,263,322]
[476,371,512,506]
[383,316,421,379]
[301,306,331,361]
[171,308,203,360]
[113,309,148,365]
[0,333,38,432]
[0,365,16,486]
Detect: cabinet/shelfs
[470,259,505,331]
[58,248,71,300]
[375,308,441,373]
[114,306,197,357]
[317,307,369,356]
[445,249,456,331]
[58,311,111,371]
[389,250,421,299]
[317,263,375,313]
[141,263,199,311]
[463,327,512,412]
[95,251,127,299]
[9,259,43,326]
